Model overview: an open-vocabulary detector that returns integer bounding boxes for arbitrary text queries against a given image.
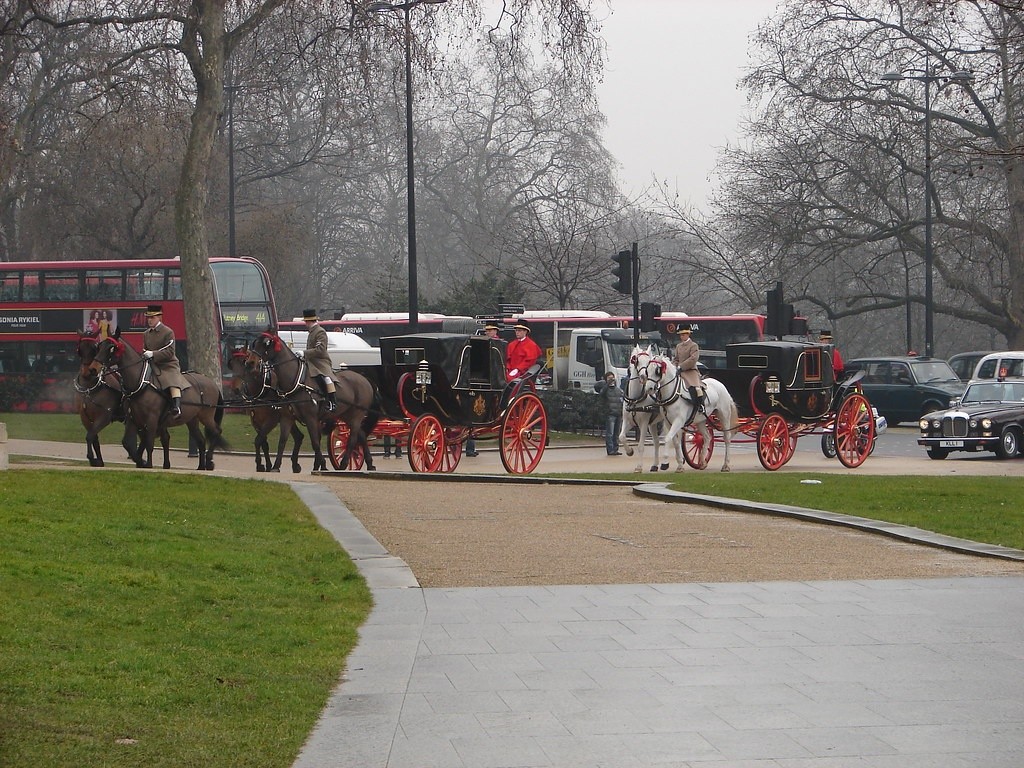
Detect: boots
[325,390,337,414]
[165,397,183,414]
[697,396,706,413]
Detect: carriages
[618,341,874,472]
[74,324,548,475]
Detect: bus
[0,255,279,413]
[278,309,807,370]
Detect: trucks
[534,322,640,394]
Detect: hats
[483,321,499,330]
[818,330,834,340]
[513,319,531,332]
[300,309,318,320]
[143,304,163,317]
[676,324,694,334]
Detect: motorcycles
[821,398,888,458]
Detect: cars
[917,350,1024,459]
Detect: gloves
[142,349,153,359]
[509,369,519,376]
[295,351,305,358]
[677,366,681,370]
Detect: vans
[844,356,967,427]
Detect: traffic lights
[610,250,631,294]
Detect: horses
[73,326,225,471]
[618,343,670,473]
[645,355,738,472]
[228,325,381,474]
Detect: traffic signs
[474,304,530,337]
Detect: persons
[599,371,625,455]
[88,309,115,345]
[482,319,542,382]
[465,439,479,457]
[816,331,843,380]
[383,434,403,459]
[674,325,704,412]
[144,304,192,413]
[843,370,867,448]
[293,309,341,411]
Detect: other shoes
[383,453,391,458]
[394,452,402,459]
[466,452,479,457]
[608,450,622,455]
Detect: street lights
[368,0,447,324]
[879,72,975,357]
